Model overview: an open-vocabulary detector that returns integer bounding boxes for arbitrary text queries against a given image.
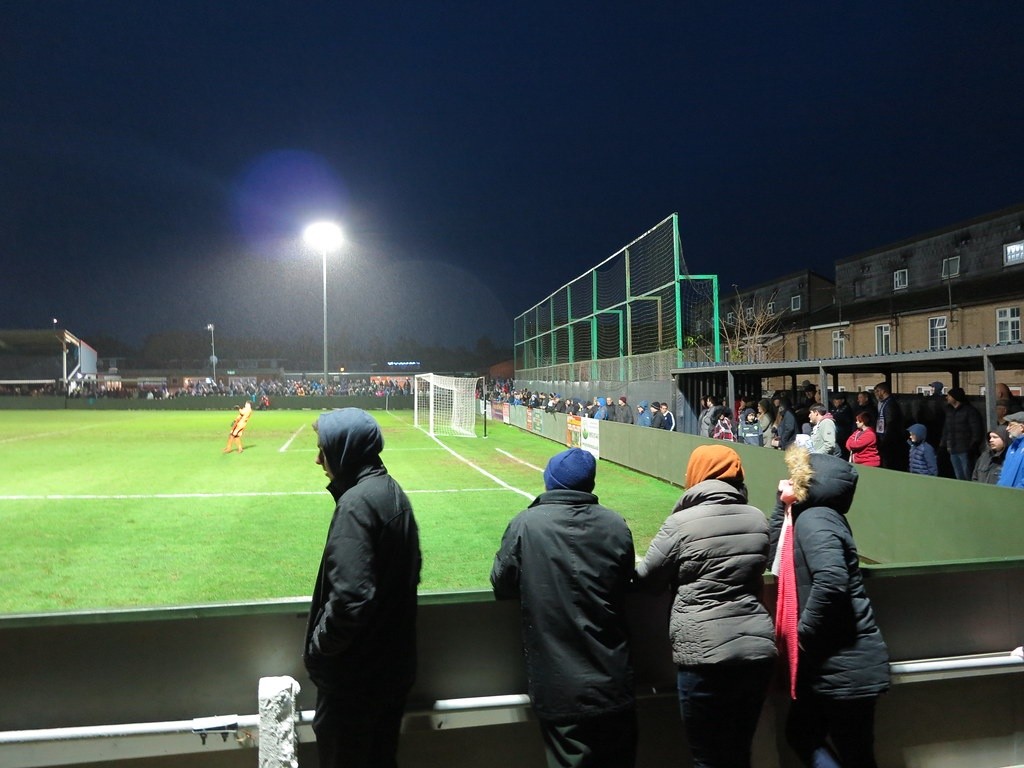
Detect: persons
[0,374,412,399]
[488,447,654,768]
[996,383,1014,427]
[766,443,892,768]
[940,388,984,482]
[906,381,948,452]
[907,424,938,476]
[634,442,773,768]
[444,371,676,432]
[972,412,1024,489]
[222,399,252,453]
[698,379,903,471]
[259,395,269,410]
[304,407,422,768]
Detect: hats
[1003,412,1024,423]
[948,388,965,402]
[620,397,626,403]
[651,402,660,410]
[543,448,596,493]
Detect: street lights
[302,221,342,396]
